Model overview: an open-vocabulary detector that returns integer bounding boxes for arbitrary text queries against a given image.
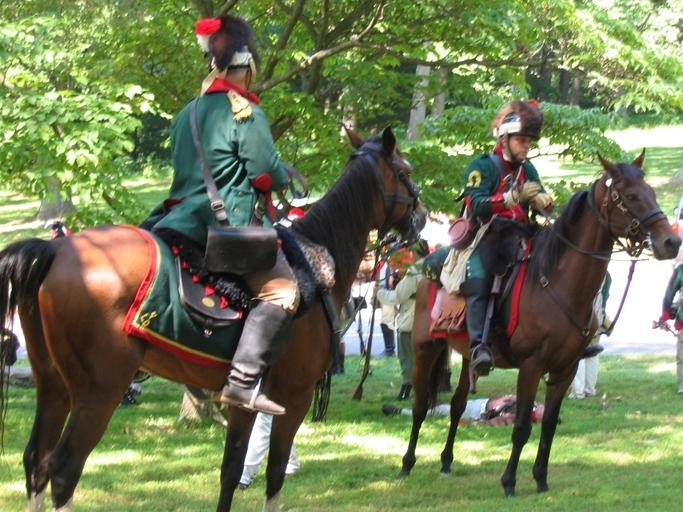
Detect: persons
[138,13,300,416]
[570,270,611,400]
[661,264,683,394]
[382,394,562,427]
[235,411,300,488]
[448,100,554,377]
[359,230,450,400]
[49,217,66,241]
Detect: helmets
[194,15,261,73]
[490,100,542,141]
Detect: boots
[377,323,396,360]
[393,383,412,401]
[439,368,451,392]
[218,299,294,415]
[459,277,491,375]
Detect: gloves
[502,179,553,211]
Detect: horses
[399,145,683,500]
[0,123,430,510]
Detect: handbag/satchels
[200,225,278,275]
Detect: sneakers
[381,404,401,416]
[567,390,596,400]
